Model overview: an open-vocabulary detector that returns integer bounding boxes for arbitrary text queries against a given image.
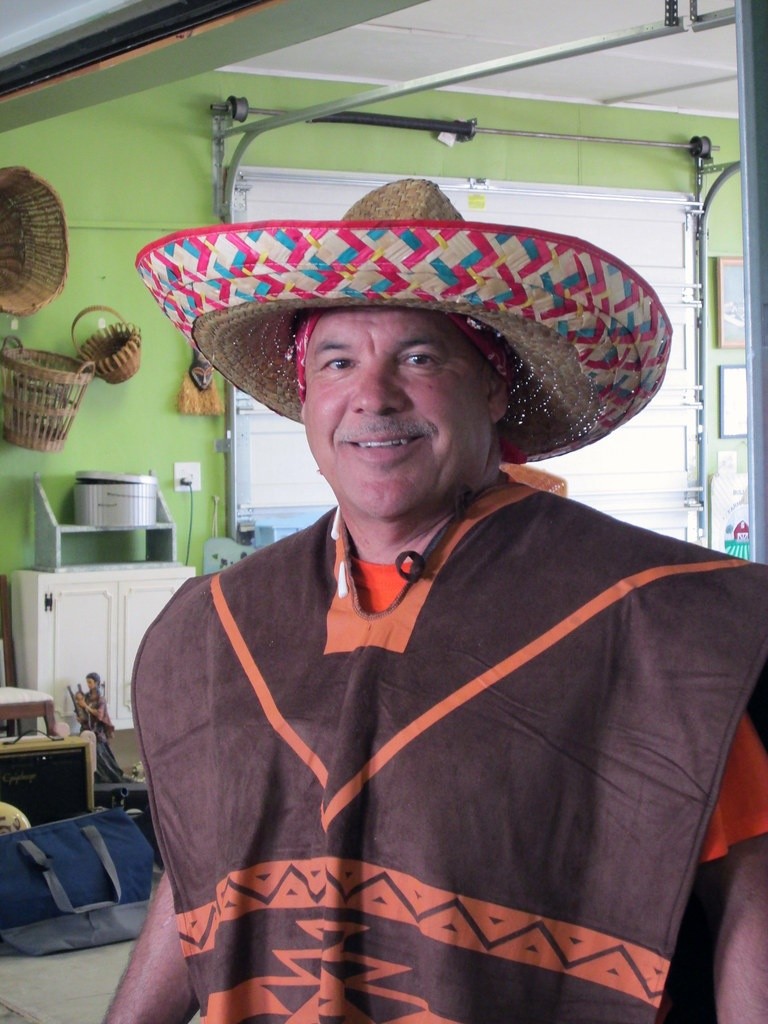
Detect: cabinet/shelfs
[11,563,195,736]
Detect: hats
[135,178,675,462]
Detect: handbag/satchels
[0,806,155,956]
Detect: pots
[73,471,158,527]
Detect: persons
[75,673,123,782]
[101,181,768,1024]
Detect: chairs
[0,574,55,736]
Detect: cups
[122,529,147,561]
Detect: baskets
[0,167,69,316]
[72,305,142,383]
[0,336,96,453]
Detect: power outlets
[172,462,202,492]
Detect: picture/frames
[719,365,747,438]
[716,255,745,348]
